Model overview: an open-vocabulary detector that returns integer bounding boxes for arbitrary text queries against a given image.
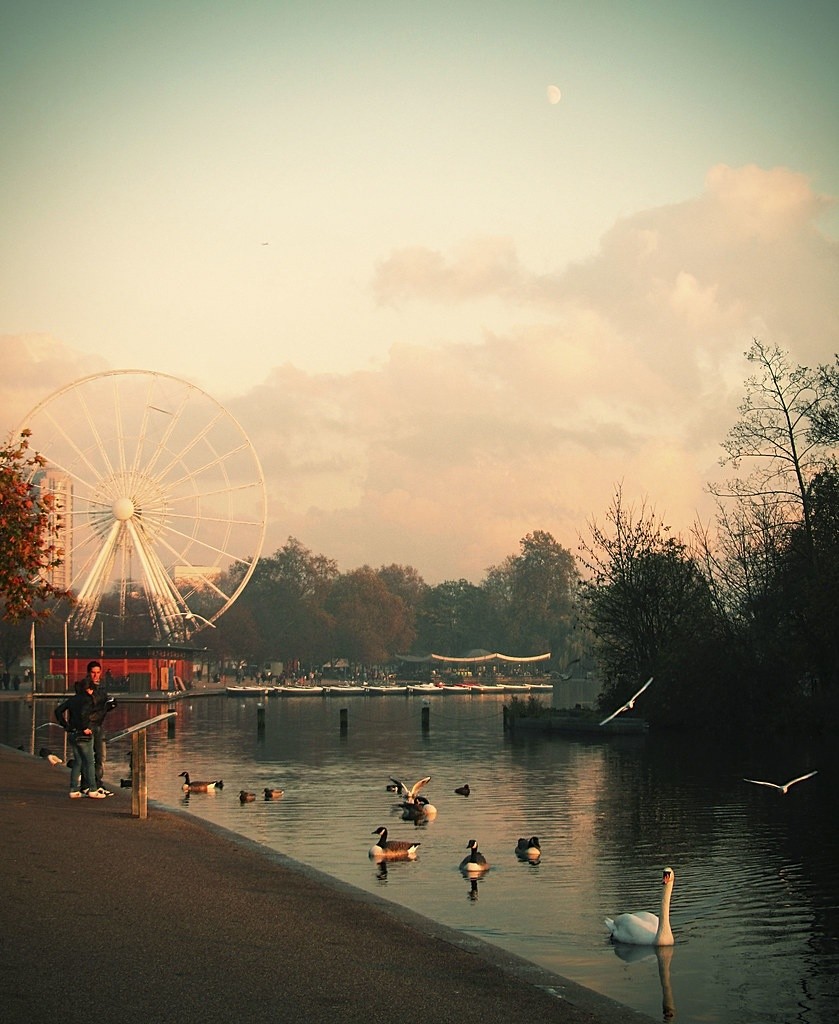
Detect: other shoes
[89,791,107,799]
[80,788,90,798]
[98,788,115,797]
[70,790,83,799]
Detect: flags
[30,626,35,648]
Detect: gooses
[515,834,542,860]
[237,790,256,804]
[604,867,675,949]
[387,775,438,829]
[177,772,224,794]
[459,838,489,873]
[368,827,421,858]
[453,784,470,796]
[262,788,285,798]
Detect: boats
[226,684,552,694]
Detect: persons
[195,660,535,686]
[0,666,33,691]
[106,668,112,693]
[80,661,117,796]
[54,679,107,799]
